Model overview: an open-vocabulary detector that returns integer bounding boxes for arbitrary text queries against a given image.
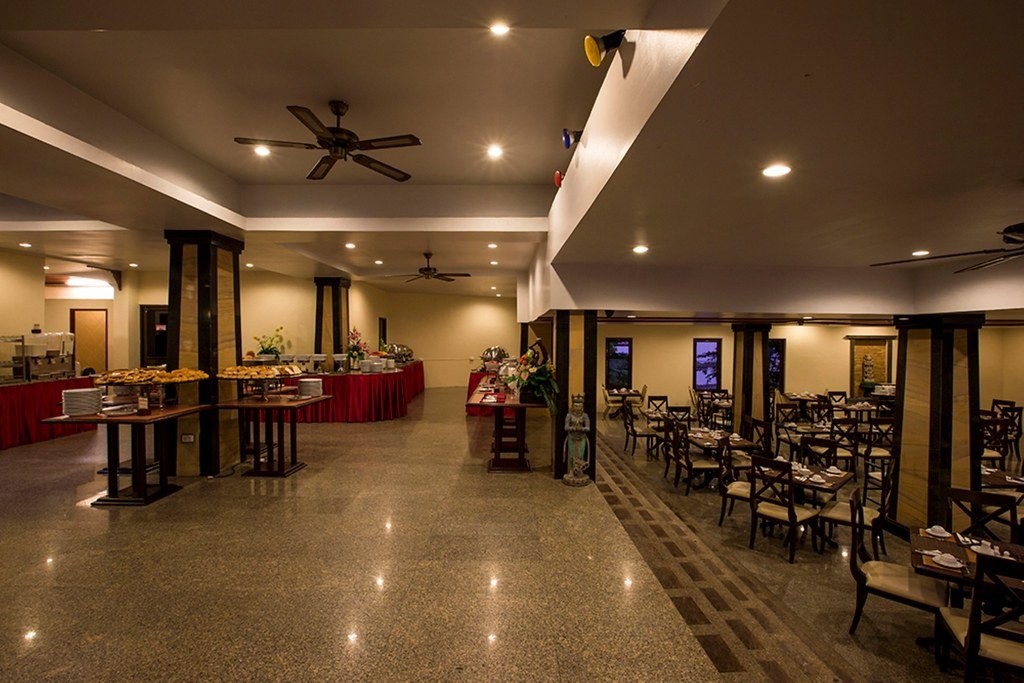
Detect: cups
[981,541,991,550]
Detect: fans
[233,101,421,183]
[867,221,1024,275]
[382,253,471,283]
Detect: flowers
[253,326,291,365]
[347,326,370,358]
[509,350,538,384]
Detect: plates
[287,395,311,400]
[692,435,702,437]
[926,528,951,537]
[730,435,740,438]
[932,554,962,567]
[360,359,382,372]
[104,409,138,415]
[809,478,825,482]
[380,359,394,369]
[298,380,322,397]
[827,468,841,473]
[62,388,101,415]
[700,430,709,432]
[101,395,138,405]
[971,545,996,556]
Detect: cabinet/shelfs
[0,335,26,384]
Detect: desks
[763,463,854,547]
[243,369,404,424]
[777,421,892,457]
[642,411,697,457]
[217,394,334,478]
[908,524,1024,653]
[699,392,732,421]
[608,390,642,420]
[712,402,732,428]
[394,360,424,404]
[465,376,548,473]
[676,429,762,490]
[783,392,819,420]
[41,404,210,507]
[782,432,891,483]
[0,375,97,451]
[841,404,891,421]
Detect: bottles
[136,387,151,416]
[31,323,41,333]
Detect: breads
[217,364,302,379]
[152,368,209,382]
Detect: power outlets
[181,434,195,443]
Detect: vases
[258,354,281,366]
[349,352,366,370]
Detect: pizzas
[94,368,154,384]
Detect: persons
[564,393,590,479]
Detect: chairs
[600,384,1024,683]
